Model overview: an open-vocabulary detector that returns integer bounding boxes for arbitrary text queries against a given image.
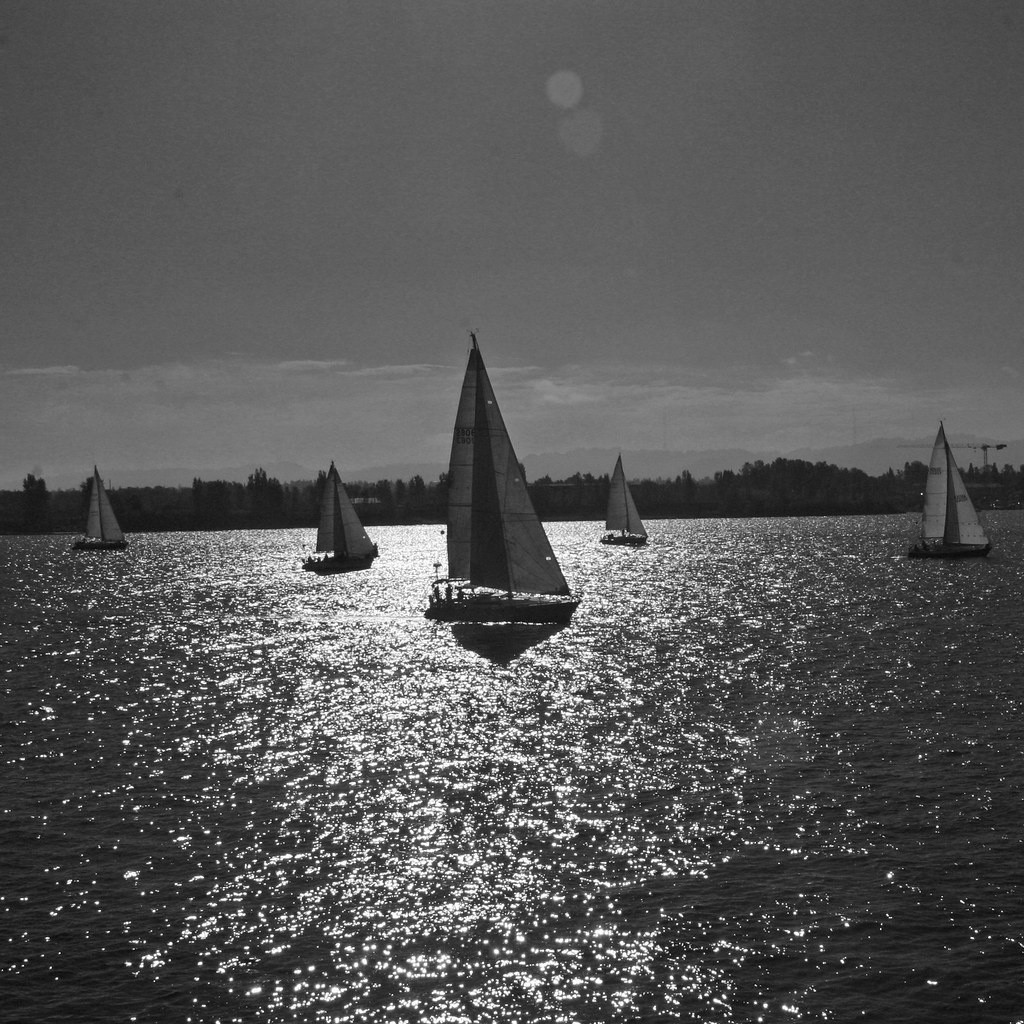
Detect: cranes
[897,442,1006,471]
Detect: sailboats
[302,459,381,576]
[600,452,650,546]
[424,328,581,626]
[73,465,129,549]
[907,421,995,559]
